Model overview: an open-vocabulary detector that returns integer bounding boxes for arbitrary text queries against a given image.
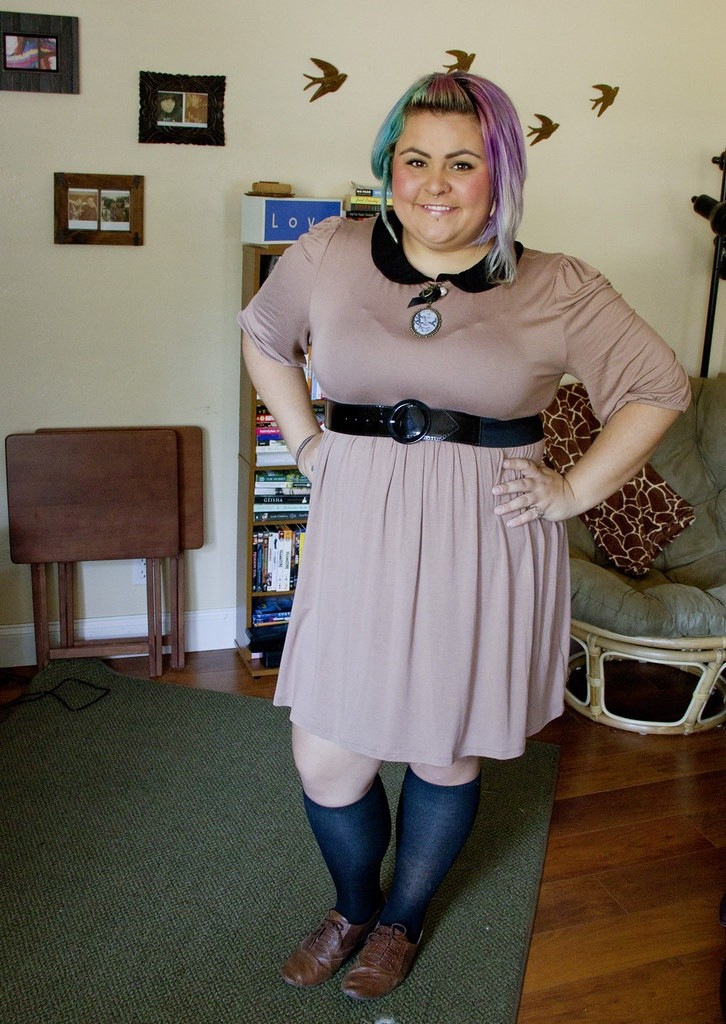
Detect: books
[252,524,307,592]
[255,406,296,465]
[343,181,393,222]
[301,344,326,400]
[252,594,294,627]
[253,470,312,520]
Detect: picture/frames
[0,10,80,95]
[138,71,226,146]
[52,172,145,246]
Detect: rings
[311,465,317,471]
[532,507,543,517]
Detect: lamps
[690,151,726,377]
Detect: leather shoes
[341,920,425,1000]
[281,892,388,988]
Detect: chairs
[539,373,726,736]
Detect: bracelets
[295,435,316,465]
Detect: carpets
[0,660,562,1024]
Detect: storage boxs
[240,195,344,244]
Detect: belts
[324,398,544,448]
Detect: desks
[5,425,204,678]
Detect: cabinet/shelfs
[235,242,325,677]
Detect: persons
[186,94,207,123]
[237,70,692,999]
[101,198,129,222]
[158,93,182,122]
[69,195,97,221]
